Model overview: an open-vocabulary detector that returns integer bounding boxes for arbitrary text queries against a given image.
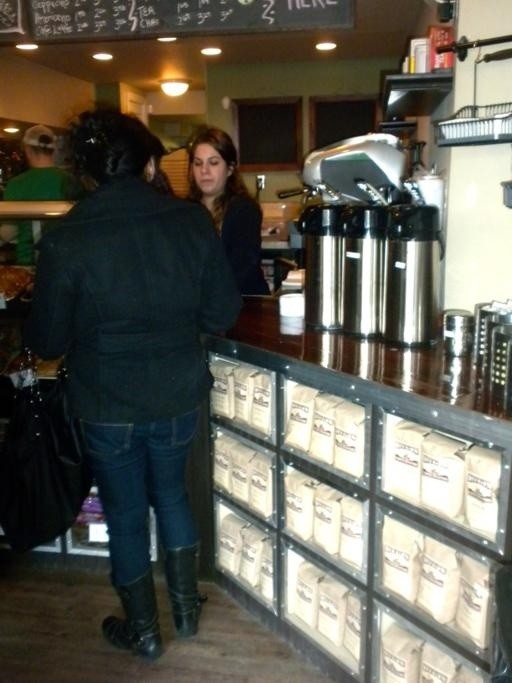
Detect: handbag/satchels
[0,374,93,552]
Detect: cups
[472,302,511,399]
[279,317,306,336]
[278,292,305,317]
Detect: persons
[184,124,272,296]
[0,124,85,266]
[21,100,243,662]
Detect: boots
[162,542,201,637]
[103,566,161,657]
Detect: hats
[24,125,58,148]
